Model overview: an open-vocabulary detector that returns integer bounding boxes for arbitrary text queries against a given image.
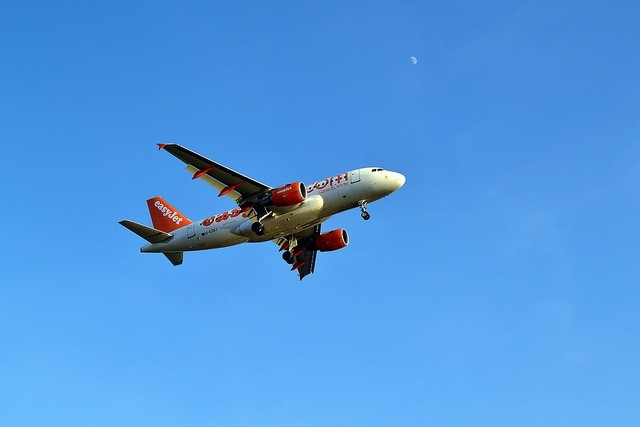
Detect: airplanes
[118,143,406,280]
